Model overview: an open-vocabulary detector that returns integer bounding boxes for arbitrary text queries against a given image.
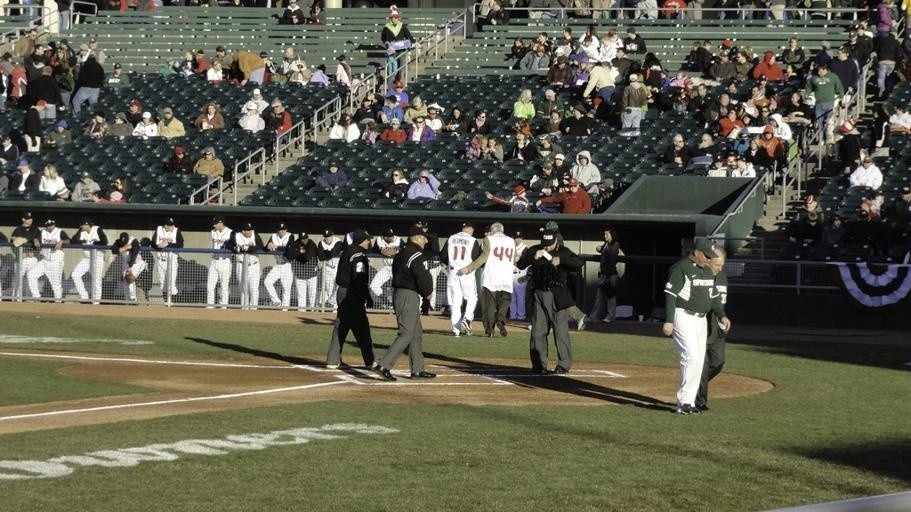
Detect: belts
[684,308,706,317]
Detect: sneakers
[488,321,507,338]
[451,319,472,337]
[578,315,587,332]
[555,365,567,374]
[411,371,436,379]
[696,403,709,411]
[326,363,349,369]
[366,363,397,381]
[677,405,701,414]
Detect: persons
[515,228,587,376]
[263,221,296,312]
[662,235,733,416]
[527,220,589,332]
[439,218,484,338]
[323,227,381,371]
[225,222,265,311]
[149,215,185,304]
[206,214,233,310]
[373,225,437,382]
[509,230,531,321]
[0,0,911,263]
[457,221,515,338]
[413,217,442,311]
[26,215,72,304]
[369,226,406,305]
[68,215,108,305]
[285,230,318,313]
[695,244,732,410]
[111,231,146,303]
[344,225,373,250]
[9,210,42,303]
[318,227,345,313]
[589,229,620,323]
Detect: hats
[239,221,254,231]
[120,233,129,243]
[275,223,334,239]
[20,212,56,226]
[541,231,555,247]
[692,236,718,259]
[211,216,222,231]
[353,230,372,238]
[569,178,578,187]
[543,160,552,169]
[82,170,92,178]
[161,217,175,225]
[383,226,395,235]
[554,153,564,161]
[77,217,93,225]
[514,186,525,194]
[164,107,172,113]
[407,228,423,234]
[142,111,151,119]
[805,193,816,204]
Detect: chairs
[0,74,911,263]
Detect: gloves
[366,296,374,309]
[420,298,433,315]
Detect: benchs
[0,1,862,74]
